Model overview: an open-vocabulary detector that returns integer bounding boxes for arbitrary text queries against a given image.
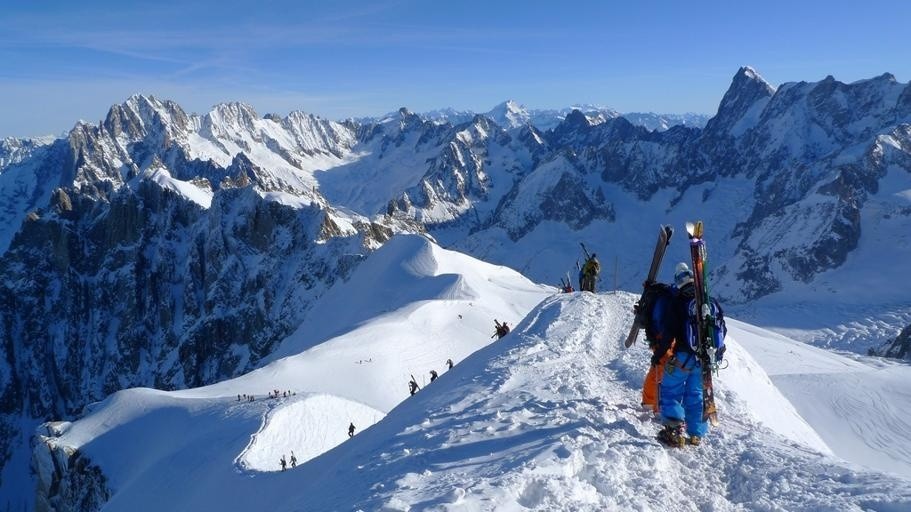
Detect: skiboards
[625,225,676,348]
[411,374,422,392]
[567,269,572,286]
[579,242,604,281]
[685,220,720,428]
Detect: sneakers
[688,435,701,447]
[656,428,687,450]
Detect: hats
[673,262,691,277]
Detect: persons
[583,253,599,291]
[446,358,454,371]
[348,422,357,438]
[651,262,727,446]
[280,458,287,472]
[409,380,418,397]
[494,325,503,338]
[501,321,511,336]
[632,278,676,415]
[578,268,584,290]
[289,455,297,468]
[429,370,438,383]
[237,388,297,402]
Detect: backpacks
[683,290,727,362]
